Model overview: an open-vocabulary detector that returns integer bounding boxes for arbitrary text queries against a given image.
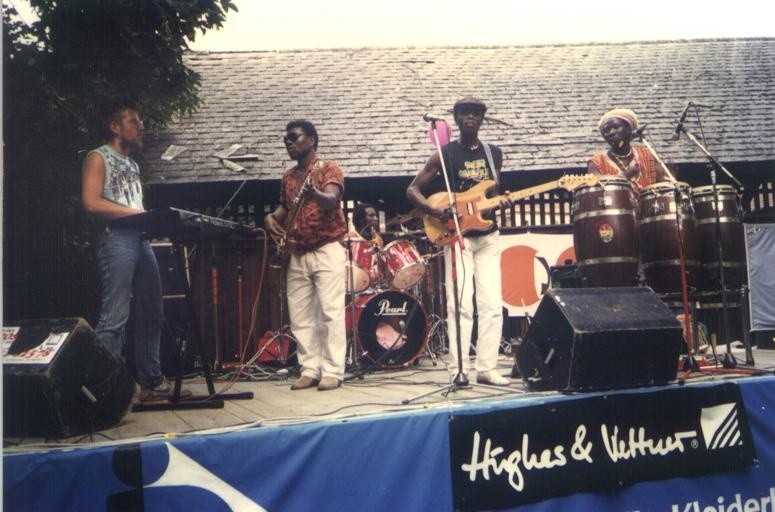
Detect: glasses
[283,132,310,144]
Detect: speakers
[2,317,137,438]
[515,286,683,392]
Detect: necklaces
[611,148,634,160]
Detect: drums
[344,236,372,297]
[692,184,744,270]
[640,181,698,269]
[378,239,428,289]
[346,288,429,371]
[573,176,640,270]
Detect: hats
[599,106,637,140]
[453,94,489,115]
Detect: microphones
[671,102,693,141]
[422,112,444,123]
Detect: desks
[662,285,756,368]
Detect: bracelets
[269,212,276,220]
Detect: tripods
[238,270,302,377]
[402,233,521,406]
[677,272,753,387]
[412,269,476,366]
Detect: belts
[289,236,329,256]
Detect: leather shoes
[290,374,317,391]
[449,372,468,386]
[317,375,343,392]
[477,368,512,387]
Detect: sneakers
[137,374,194,403]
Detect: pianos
[110,206,257,244]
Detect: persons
[343,202,384,250]
[78,95,193,405]
[263,119,351,392]
[404,95,517,389]
[586,108,666,189]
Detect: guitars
[275,159,324,265]
[423,173,598,246]
